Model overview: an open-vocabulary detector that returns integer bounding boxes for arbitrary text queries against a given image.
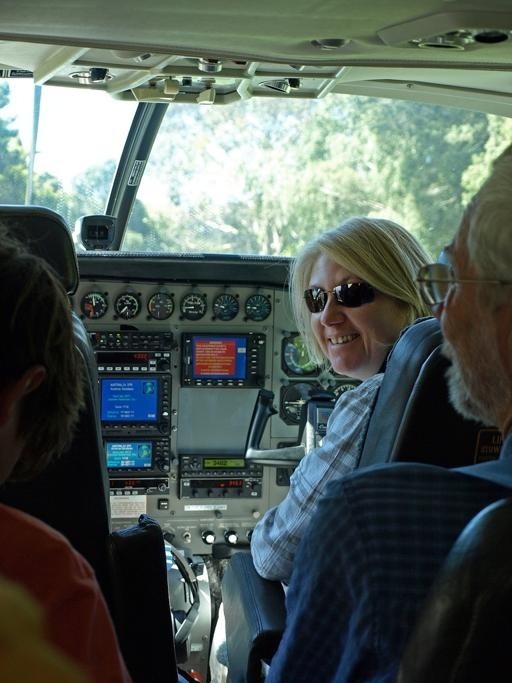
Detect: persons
[249,217,438,592]
[266,142,512,683]
[0,219,133,683]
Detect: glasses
[303,280,380,313]
[416,262,497,306]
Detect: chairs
[221,314,504,680]
[1,202,201,683]
